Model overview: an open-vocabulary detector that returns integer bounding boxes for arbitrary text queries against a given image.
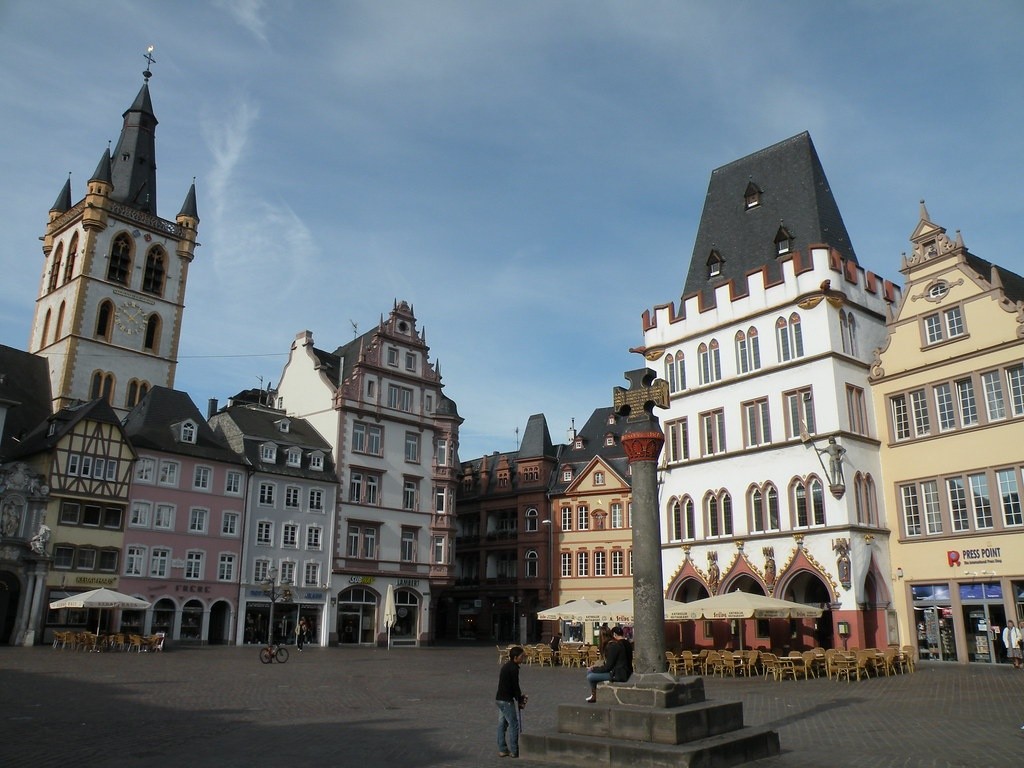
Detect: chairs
[496,643,916,683]
[51,629,165,654]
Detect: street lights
[541,520,552,597]
[261,567,291,656]
[509,597,523,643]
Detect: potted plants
[454,576,462,590]
[463,576,472,590]
[472,574,482,590]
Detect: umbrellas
[48,586,152,636]
[537,597,604,647]
[664,589,823,650]
[384,584,397,651]
[573,599,689,622]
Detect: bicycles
[260,641,289,663]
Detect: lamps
[837,620,849,638]
[897,567,903,576]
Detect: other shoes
[586,692,596,703]
[1014,662,1019,669]
[510,752,519,758]
[298,648,302,651]
[499,751,510,757]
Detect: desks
[815,654,824,674]
[876,653,885,675]
[721,655,743,674]
[578,650,586,666]
[777,657,802,679]
[681,654,700,673]
[898,650,908,674]
[831,656,855,678]
[141,637,152,652]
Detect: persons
[245,613,314,652]
[586,623,633,703]
[30,526,51,557]
[816,436,846,485]
[550,632,563,665]
[1002,620,1024,669]
[495,647,528,757]
[391,621,407,635]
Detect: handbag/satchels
[592,660,605,667]
[1017,639,1024,650]
